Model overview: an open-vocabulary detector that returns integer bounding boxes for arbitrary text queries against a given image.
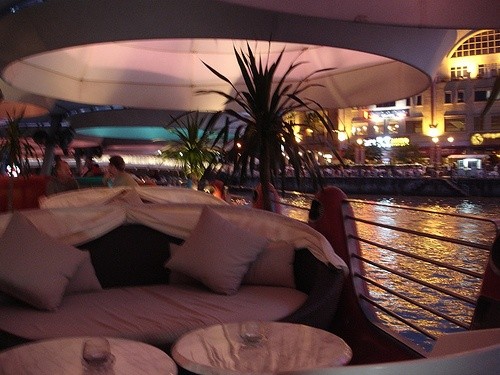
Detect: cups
[84,339,110,368]
[239,320,266,345]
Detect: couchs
[0,203,344,344]
[1,176,231,209]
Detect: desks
[172,322,352,375]
[1,337,178,375]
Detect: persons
[79,157,108,178]
[127,166,235,187]
[108,155,138,187]
[46,160,79,194]
[285,162,459,176]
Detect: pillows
[164,205,269,296]
[1,213,90,313]
